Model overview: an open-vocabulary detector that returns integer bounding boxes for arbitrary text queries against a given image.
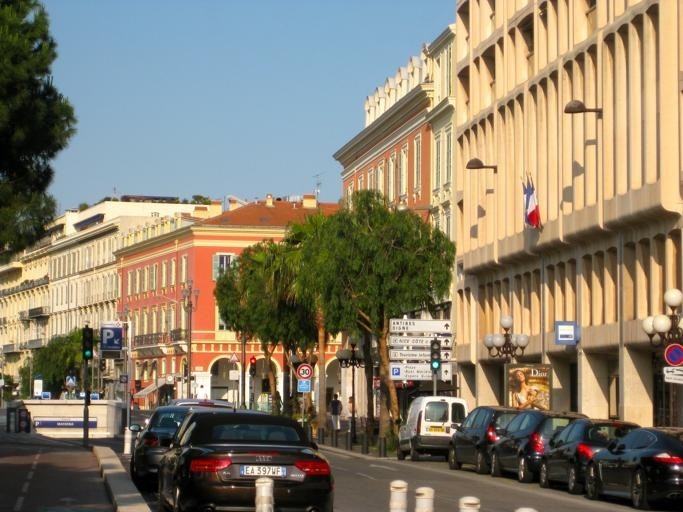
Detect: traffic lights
[82,328,93,360]
[184,364,194,383]
[99,358,106,371]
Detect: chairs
[219,429,288,441]
[160,416,175,427]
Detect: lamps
[564,100,602,113]
[466,158,497,173]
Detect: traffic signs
[249,354,256,377]
[388,318,454,382]
[297,363,314,379]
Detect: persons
[345,396,359,432]
[509,370,548,412]
[273,390,284,414]
[329,393,343,432]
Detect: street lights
[335,337,365,443]
[642,288,683,427]
[485,315,530,363]
[181,277,200,398]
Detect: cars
[129,398,335,512]
[448,406,682,509]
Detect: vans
[397,396,468,461]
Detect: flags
[520,170,540,228]
